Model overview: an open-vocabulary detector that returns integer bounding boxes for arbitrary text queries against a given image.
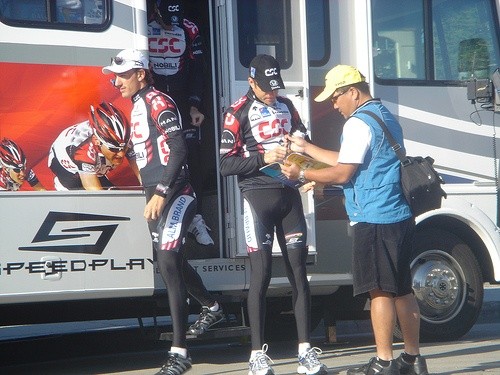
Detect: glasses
[331,87,351,103]
[110,57,144,65]
[107,147,126,152]
[13,169,25,173]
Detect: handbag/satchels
[400,156,446,216]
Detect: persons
[147,0,214,245]
[102,47,224,375]
[0,138,46,191]
[218,54,328,375]
[47,100,142,190]
[283,64,428,375]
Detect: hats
[158,0,184,25]
[248,54,285,91]
[313,65,366,102]
[102,50,149,74]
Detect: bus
[0,0,500,344]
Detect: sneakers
[347,353,429,375]
[248,343,274,375]
[154,351,192,375]
[297,347,328,375]
[191,301,225,334]
[187,214,215,246]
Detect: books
[258,152,333,192]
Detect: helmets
[88,101,130,149]
[0,137,26,169]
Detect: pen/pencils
[287,139,291,150]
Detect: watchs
[298,168,306,183]
